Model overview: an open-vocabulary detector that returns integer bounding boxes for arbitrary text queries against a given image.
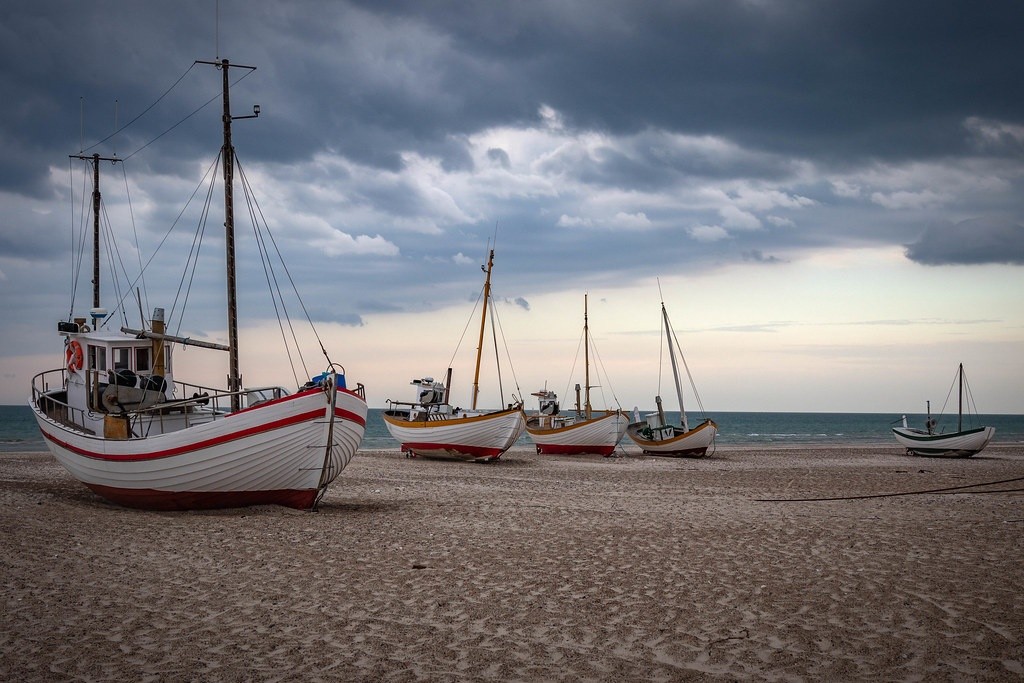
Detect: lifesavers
[66,341,83,374]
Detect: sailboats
[894,359,998,457]
[28,56,369,510]
[517,294,630,461]
[380,240,529,464]
[624,301,721,454]
